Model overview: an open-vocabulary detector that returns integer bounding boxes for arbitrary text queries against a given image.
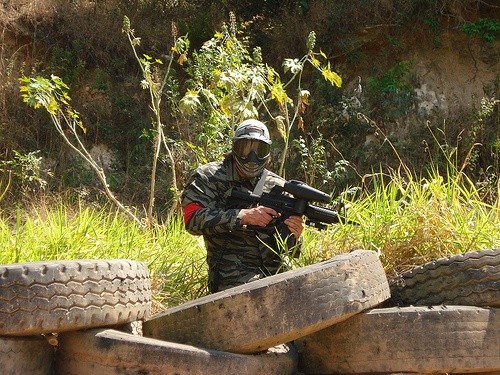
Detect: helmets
[231,119,272,177]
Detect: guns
[226,179,360,250]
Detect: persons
[180,119,305,295]
